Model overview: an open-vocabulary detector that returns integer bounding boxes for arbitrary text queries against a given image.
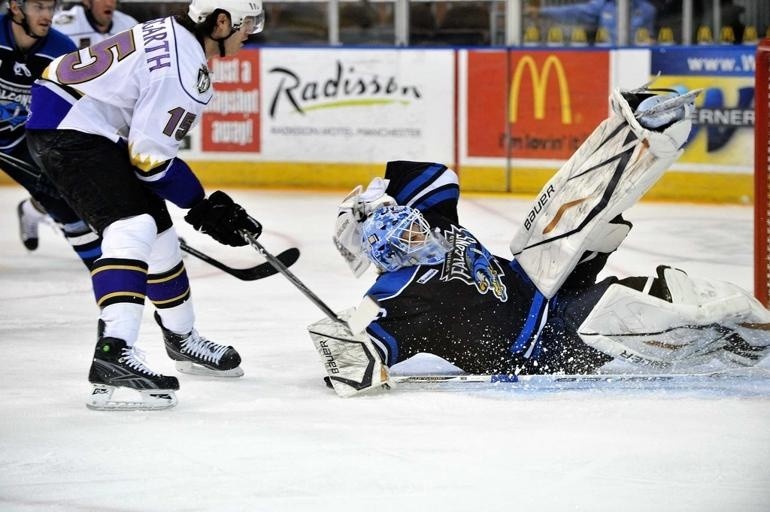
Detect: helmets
[361,207,456,273]
[187,1,265,36]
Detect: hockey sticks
[229,223,381,336]
[179,242,298,282]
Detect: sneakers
[166,329,241,370]
[88,337,179,390]
[18,199,39,250]
[637,94,684,132]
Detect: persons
[408,1,492,46]
[308,68,770,399]
[525,1,657,46]
[18,0,140,251]
[26,0,265,412]
[0,0,102,272]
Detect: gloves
[184,191,261,246]
